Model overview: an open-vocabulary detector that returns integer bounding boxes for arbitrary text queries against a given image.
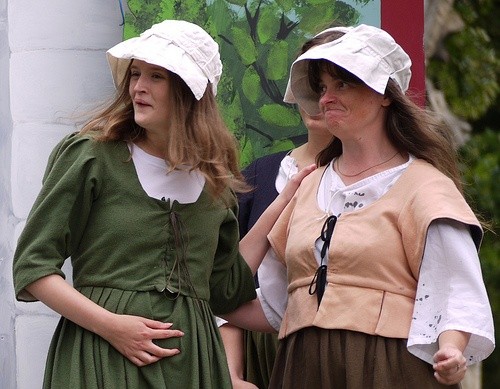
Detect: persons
[220,24,495,389]
[214,26,353,389]
[13,19,317,389]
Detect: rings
[457,364,459,370]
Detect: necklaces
[337,152,400,176]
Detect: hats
[104,19,223,103]
[285,24,413,120]
[279,26,354,104]
